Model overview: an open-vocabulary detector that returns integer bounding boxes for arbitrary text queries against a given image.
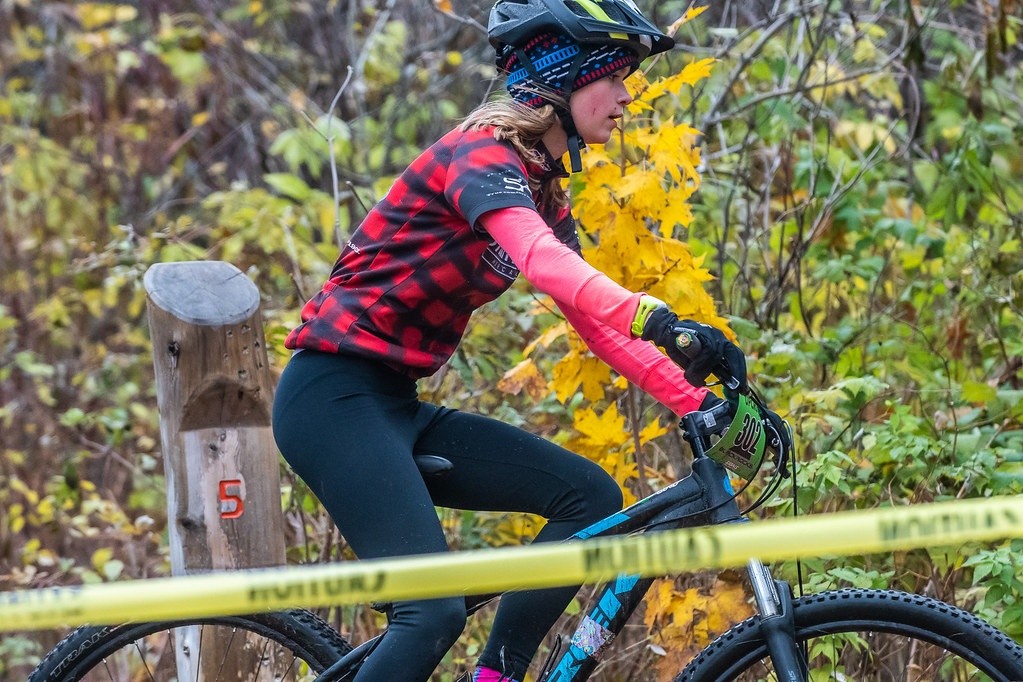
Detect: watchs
[631,295,668,337]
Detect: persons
[271,0,790,682]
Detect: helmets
[486,0,676,62]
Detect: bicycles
[23,332,1023,682]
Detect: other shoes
[473,665,518,682]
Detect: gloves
[641,308,747,398]
[698,391,790,463]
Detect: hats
[505,30,637,106]
